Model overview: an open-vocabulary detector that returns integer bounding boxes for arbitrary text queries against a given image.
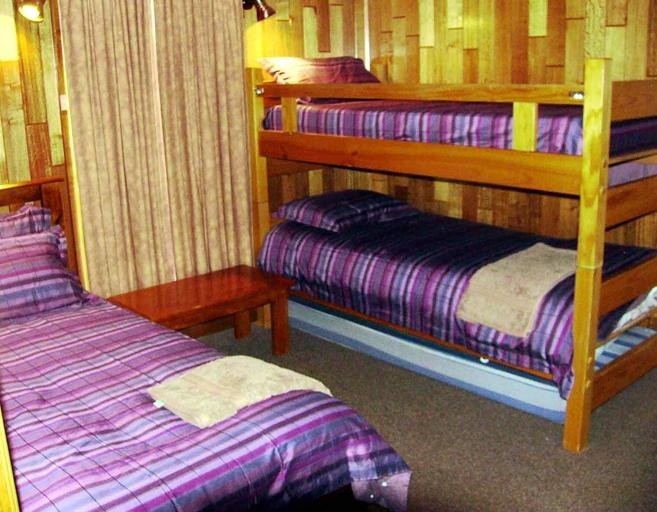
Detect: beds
[2,179,412,511]
[246,57,656,199]
[247,198,656,454]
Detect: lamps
[240,0,277,22]
[17,0,46,24]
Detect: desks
[105,264,295,357]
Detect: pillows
[262,57,381,105]
[1,204,51,238]
[1,224,104,325]
[271,187,422,232]
